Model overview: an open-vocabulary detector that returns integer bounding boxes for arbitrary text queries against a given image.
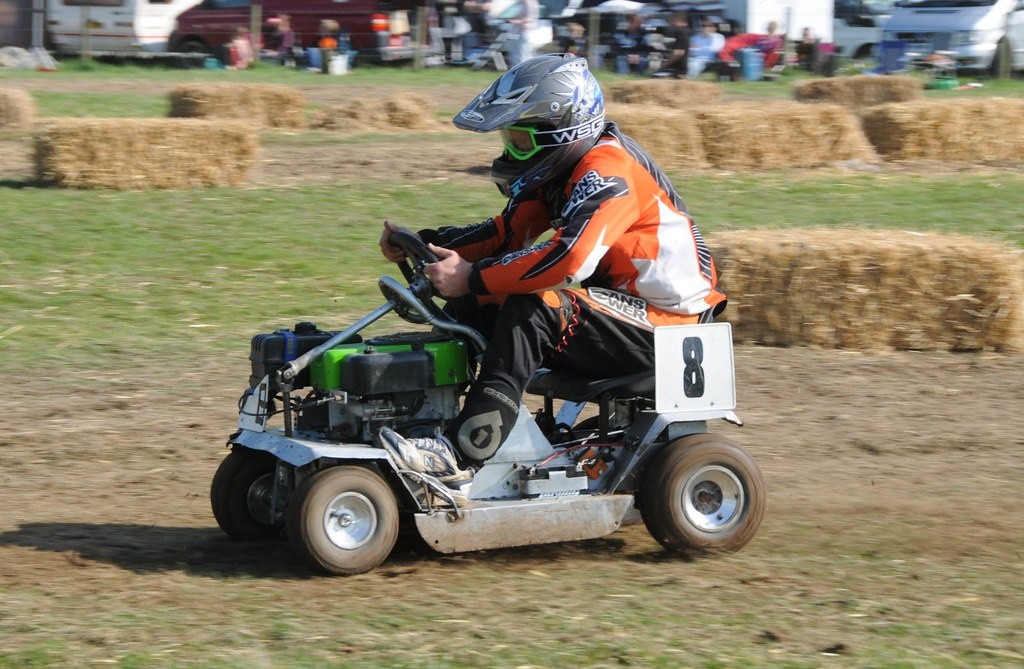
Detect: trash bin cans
[737,47,766,81]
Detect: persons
[228,14,340,70]
[561,12,726,79]
[379,52,728,508]
[755,20,821,71]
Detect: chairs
[525,302,716,445]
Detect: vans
[835,0,897,59]
[882,0,1024,79]
[168,0,446,68]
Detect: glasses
[503,112,604,160]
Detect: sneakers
[371,426,476,507]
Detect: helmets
[453,54,604,199]
